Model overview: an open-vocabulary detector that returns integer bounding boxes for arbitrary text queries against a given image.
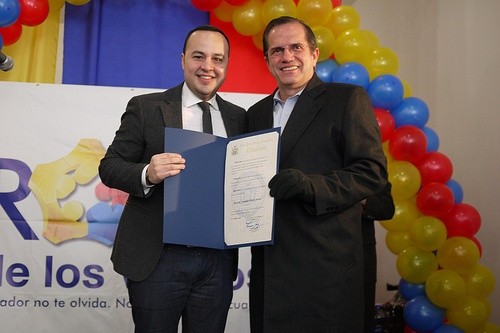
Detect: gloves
[268,168,314,202]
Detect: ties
[197,102,213,135]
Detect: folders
[162,127,281,250]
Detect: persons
[98,25,246,333]
[246,16,388,332]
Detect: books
[163,126,281,249]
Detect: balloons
[0,0,90,53]
[192,1,500,332]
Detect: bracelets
[146,168,157,186]
